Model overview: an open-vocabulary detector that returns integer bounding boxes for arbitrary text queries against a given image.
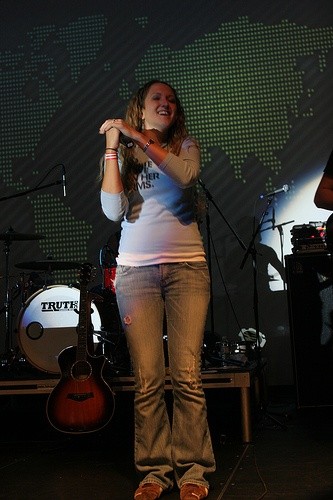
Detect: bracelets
[106,153,118,160]
[106,148,118,152]
[143,139,154,152]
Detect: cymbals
[0,231,48,240]
[14,258,85,270]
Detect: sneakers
[134,483,163,500]
[180,483,207,500]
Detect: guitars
[45,261,116,433]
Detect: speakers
[285,252,333,408]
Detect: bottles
[220,335,231,368]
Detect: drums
[16,282,121,377]
[100,263,117,294]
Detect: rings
[113,120,115,123]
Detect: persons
[98,80,217,500]
[314,149,333,210]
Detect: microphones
[62,166,67,197]
[266,184,288,197]
